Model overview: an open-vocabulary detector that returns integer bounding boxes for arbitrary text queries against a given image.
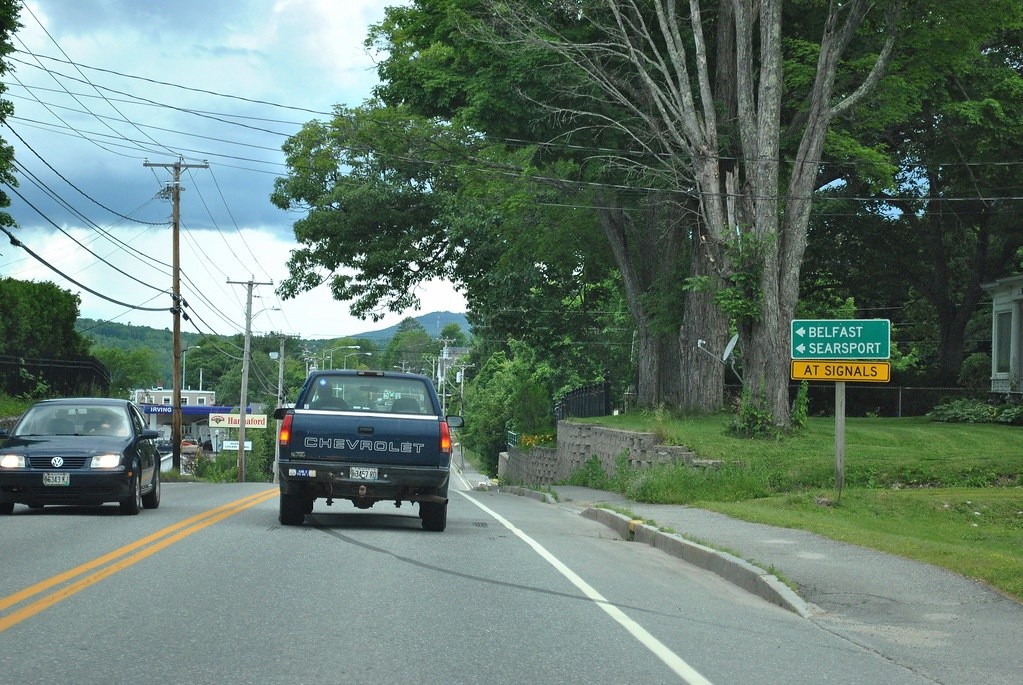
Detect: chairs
[45,418,74,434]
[310,397,347,411]
[391,398,420,415]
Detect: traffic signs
[790,319,891,360]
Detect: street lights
[394,365,404,374]
[213,430,220,457]
[239,307,282,483]
[343,352,372,370]
[182,345,201,390]
[422,369,435,386]
[330,345,360,370]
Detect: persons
[88,413,128,437]
[302,382,333,409]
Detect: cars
[203,439,212,451]
[179,437,201,455]
[0,396,162,515]
[152,436,174,457]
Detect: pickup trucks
[275,368,465,533]
